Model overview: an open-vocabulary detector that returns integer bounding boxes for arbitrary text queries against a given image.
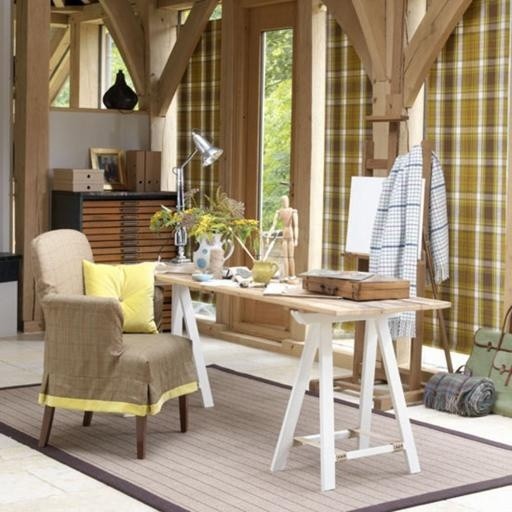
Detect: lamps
[163,133,223,264]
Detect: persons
[103,157,111,181]
[266,194,300,283]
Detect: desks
[148,259,453,492]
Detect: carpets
[1,362,512,512]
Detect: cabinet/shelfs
[51,190,178,331]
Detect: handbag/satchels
[465,306,512,420]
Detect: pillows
[83,257,160,337]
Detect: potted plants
[149,194,258,273]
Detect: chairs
[30,229,196,460]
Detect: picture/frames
[87,147,129,191]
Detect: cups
[252,261,279,284]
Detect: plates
[193,274,212,281]
